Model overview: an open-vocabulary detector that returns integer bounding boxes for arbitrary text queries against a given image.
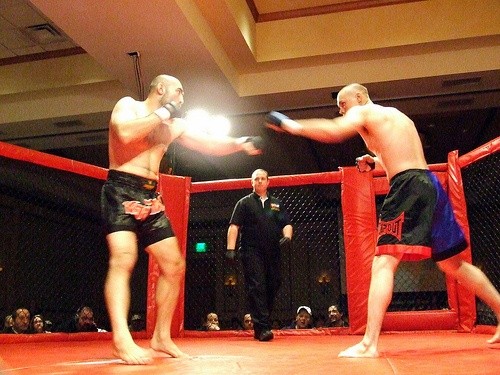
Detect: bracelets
[155,106,171,121]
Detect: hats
[297,306,312,315]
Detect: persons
[2,305,349,334]
[265,83,500,357]
[226,169,293,341]
[102,74,264,365]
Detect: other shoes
[258,328,274,342]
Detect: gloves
[279,237,291,249]
[223,249,239,270]
